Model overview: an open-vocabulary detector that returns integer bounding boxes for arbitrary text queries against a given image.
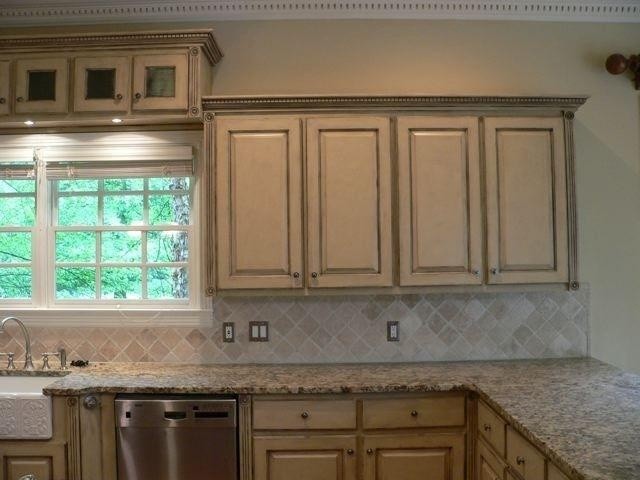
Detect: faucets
[1,315,34,371]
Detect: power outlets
[222,322,234,343]
[386,320,400,341]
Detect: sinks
[0,374,54,442]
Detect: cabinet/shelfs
[0,395,81,480]
[0,28,71,135]
[395,95,587,294]
[200,94,396,300]
[238,392,470,480]
[71,28,224,129]
[470,395,570,480]
